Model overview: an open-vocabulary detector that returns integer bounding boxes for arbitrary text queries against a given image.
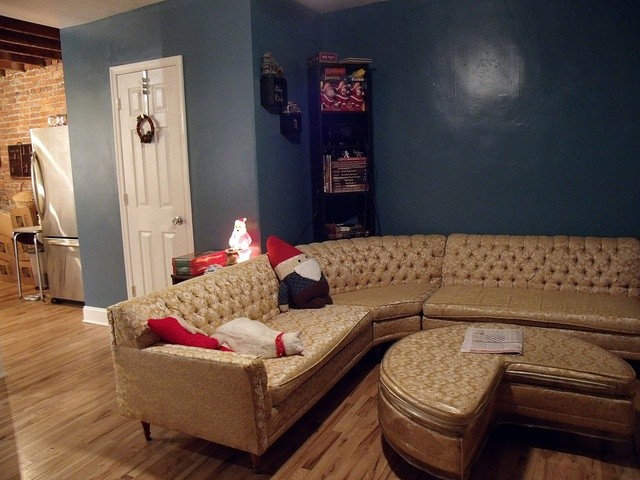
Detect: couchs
[105,233,639,473]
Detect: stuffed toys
[322,81,368,112]
[265,235,333,312]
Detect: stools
[13,225,45,302]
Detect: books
[324,155,370,193]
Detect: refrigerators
[30,125,86,305]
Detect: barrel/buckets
[28,248,48,289]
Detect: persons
[228,217,252,250]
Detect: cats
[214,316,306,358]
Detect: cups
[48,116,57,126]
[56,114,66,126]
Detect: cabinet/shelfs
[171,273,201,285]
[307,61,373,241]
